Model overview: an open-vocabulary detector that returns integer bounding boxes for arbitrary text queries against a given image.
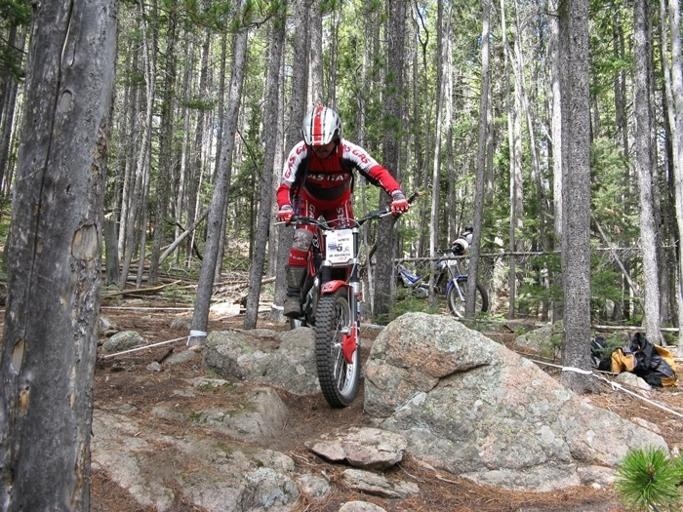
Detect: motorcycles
[273,206,402,410]
[394,225,488,318]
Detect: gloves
[391,190,409,215]
[276,205,294,227]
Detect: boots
[283,267,307,316]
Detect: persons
[272,102,412,319]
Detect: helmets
[302,106,342,147]
[452,238,468,254]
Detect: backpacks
[591,332,679,388]
